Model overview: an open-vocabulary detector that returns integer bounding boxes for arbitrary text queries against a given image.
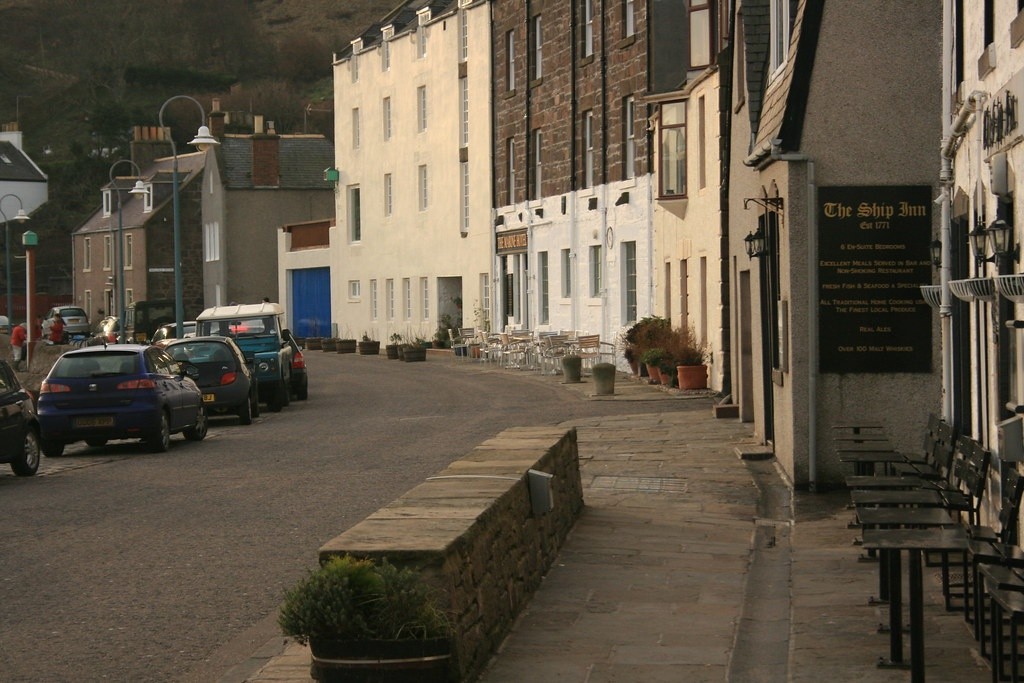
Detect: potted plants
[623,315,715,388]
[292,331,426,362]
[275,550,464,683]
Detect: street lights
[0,192,32,338]
[109,159,151,342]
[157,95,223,344]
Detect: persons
[10,322,27,371]
[49,314,65,345]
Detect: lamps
[743,225,768,260]
[964,216,996,264]
[928,231,943,268]
[985,208,1020,263]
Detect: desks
[828,422,970,683]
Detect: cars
[0,358,42,478]
[150,297,308,426]
[89,315,122,347]
[0,316,16,336]
[40,305,91,339]
[36,343,210,458]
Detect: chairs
[445,326,617,379]
[896,411,1024,683]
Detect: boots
[12,360,20,372]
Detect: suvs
[114,297,186,345]
